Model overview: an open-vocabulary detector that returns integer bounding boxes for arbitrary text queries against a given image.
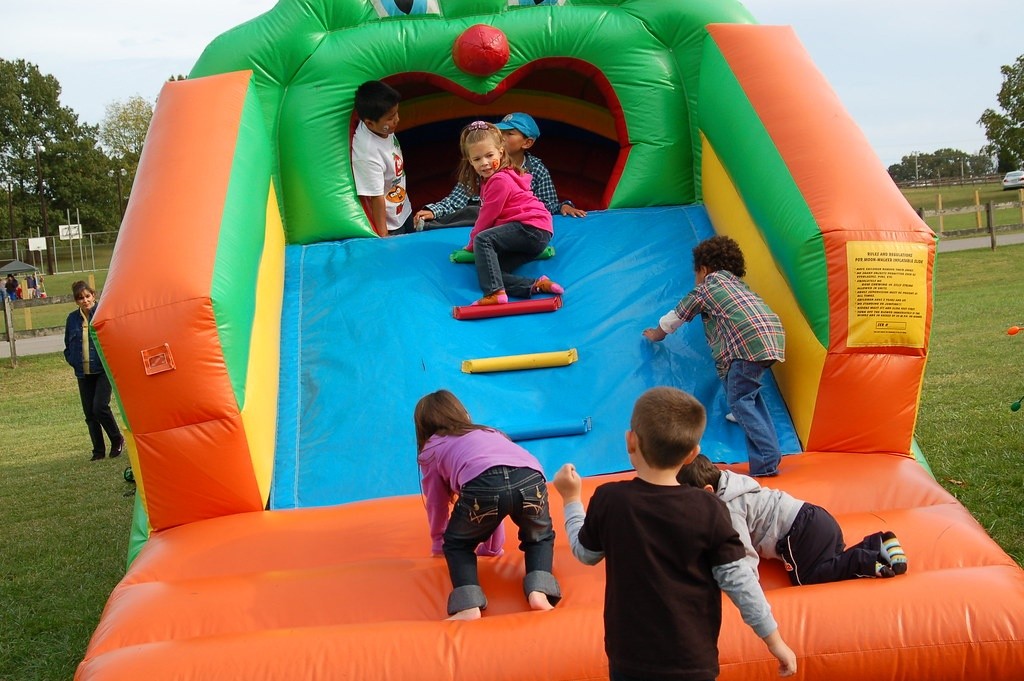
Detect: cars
[1002,171,1024,191]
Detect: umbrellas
[0,260,39,275]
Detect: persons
[0,273,39,302]
[553,385,798,681]
[351,80,414,237]
[460,121,564,305]
[414,389,562,621]
[675,454,907,587]
[63,280,124,462]
[413,112,588,232]
[641,235,786,477]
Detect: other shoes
[90,453,105,461]
[109,436,124,458]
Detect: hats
[494,112,540,140]
